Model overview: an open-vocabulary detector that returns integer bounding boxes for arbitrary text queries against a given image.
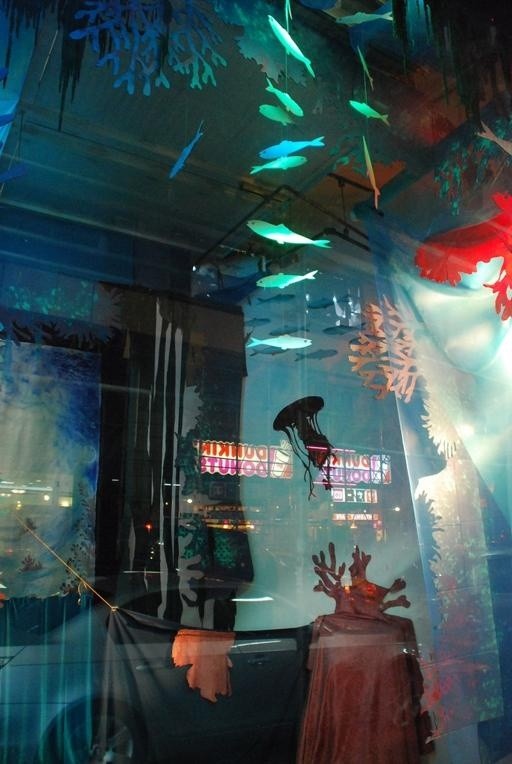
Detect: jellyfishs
[273,395,339,501]
